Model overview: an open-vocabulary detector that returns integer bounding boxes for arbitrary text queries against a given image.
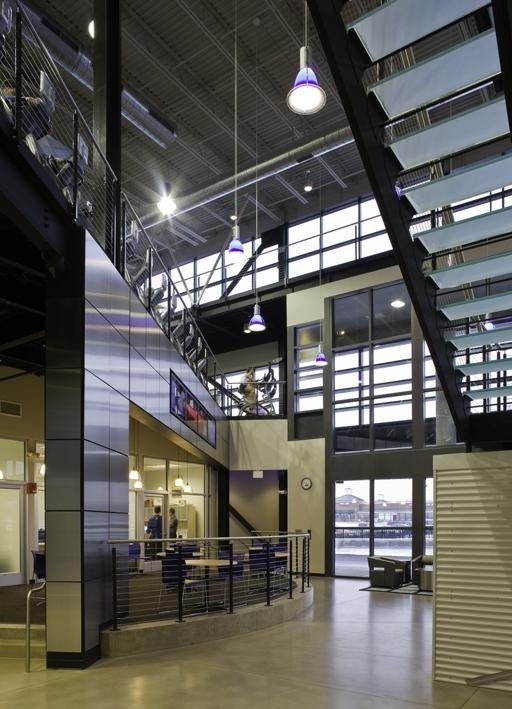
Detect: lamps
[228,1,326,365]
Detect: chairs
[129,542,154,575]
[410,554,433,583]
[162,539,289,617]
[29,528,45,607]
[368,556,406,589]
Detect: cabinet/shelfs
[144,504,196,541]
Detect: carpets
[359,581,433,596]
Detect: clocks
[300,478,312,490]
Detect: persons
[146,506,162,561]
[169,507,177,538]
[238,367,257,401]
